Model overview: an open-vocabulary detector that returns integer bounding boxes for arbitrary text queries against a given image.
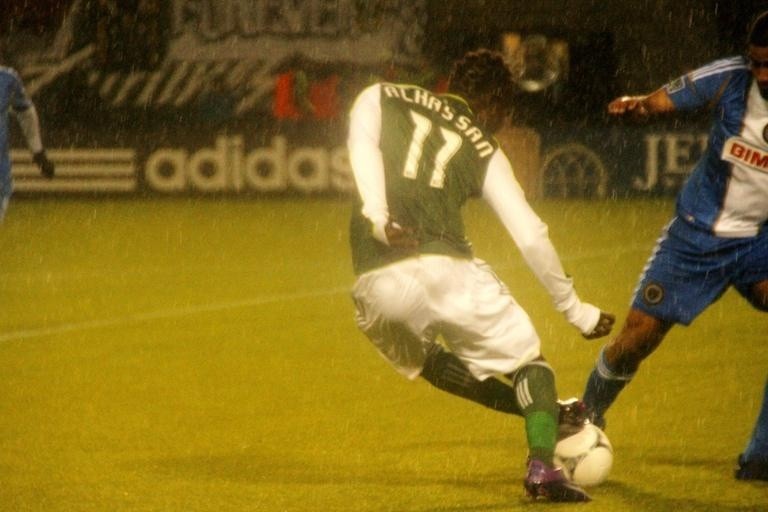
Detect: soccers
[553,423,614,486]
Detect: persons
[563,8,767,483]
[348,46,615,503]
[0,65,55,228]
[166,50,453,148]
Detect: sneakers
[735,454,768,481]
[524,458,591,503]
[562,398,606,430]
